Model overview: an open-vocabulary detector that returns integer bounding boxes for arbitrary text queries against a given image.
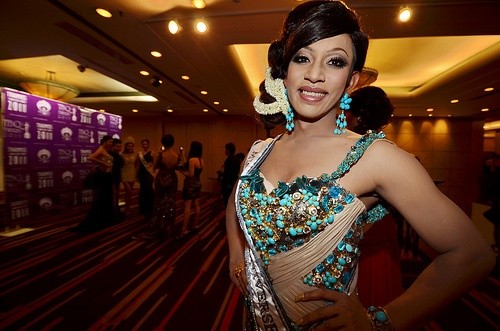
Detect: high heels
[191,226,200,235]
[175,230,189,241]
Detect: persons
[226,0,496,331]
[478,152,500,245]
[86,134,245,241]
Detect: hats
[124,137,135,145]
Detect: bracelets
[367,306,392,331]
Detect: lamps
[19,71,80,101]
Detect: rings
[234,267,245,279]
